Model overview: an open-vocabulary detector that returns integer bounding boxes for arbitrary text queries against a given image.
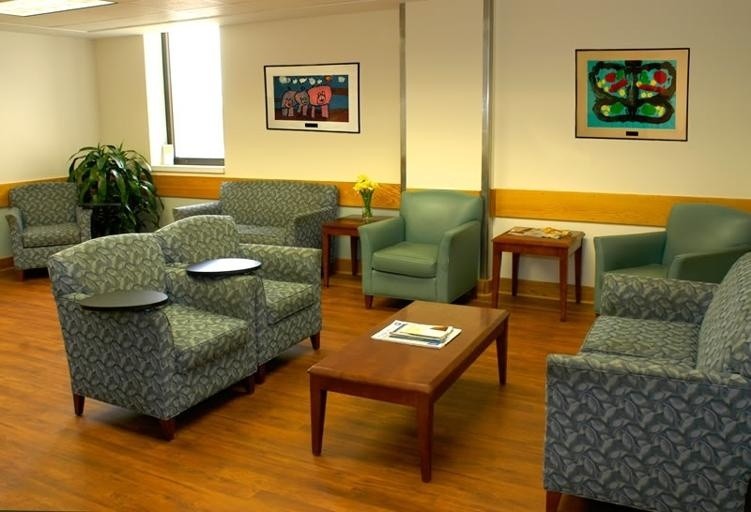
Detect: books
[542,229,572,239]
[390,322,455,343]
[527,226,553,238]
[370,319,463,350]
[508,225,532,236]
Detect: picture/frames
[262,62,360,135]
[573,46,690,142]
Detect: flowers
[352,174,378,193]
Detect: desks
[491,225,584,322]
[322,213,390,287]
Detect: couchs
[171,177,339,277]
[357,189,485,310]
[152,213,322,385]
[5,182,92,280]
[591,201,749,316]
[47,233,256,441]
[538,250,749,511]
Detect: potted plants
[69,144,162,237]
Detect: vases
[358,190,374,220]
